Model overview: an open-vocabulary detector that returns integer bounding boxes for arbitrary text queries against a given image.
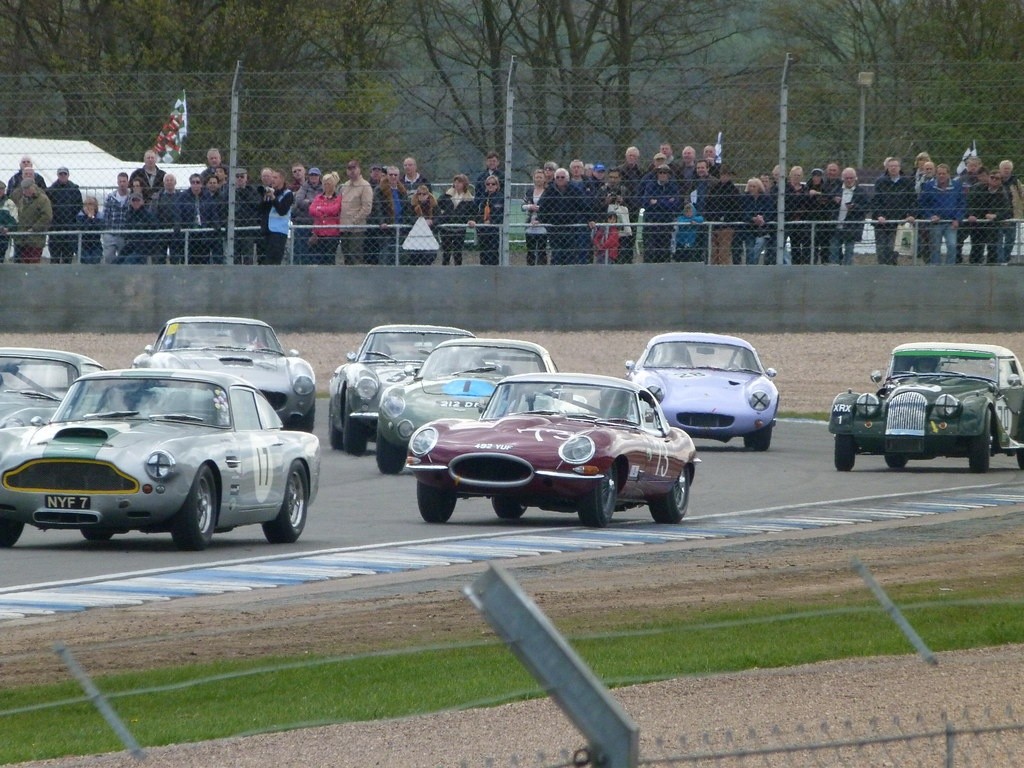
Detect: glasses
[59,173,67,176]
[486,183,495,186]
[236,175,244,178]
[293,169,301,173]
[191,180,201,184]
[556,176,566,179]
[417,193,426,195]
[22,161,30,163]
[132,199,140,202]
[388,174,399,176]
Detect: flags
[957,140,976,175]
[714,132,723,163]
[155,89,187,163]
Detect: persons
[1,144,1023,266]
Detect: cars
[129,315,317,430]
[377,338,561,474]
[826,341,1024,474]
[327,325,479,459]
[0,346,107,458]
[404,373,703,527]
[0,367,324,552]
[623,333,780,450]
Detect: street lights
[857,70,875,166]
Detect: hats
[812,169,823,177]
[347,160,358,168]
[236,168,247,174]
[57,167,69,174]
[657,164,670,173]
[0,181,6,188]
[307,168,321,175]
[21,178,35,188]
[131,192,142,200]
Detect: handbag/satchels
[608,204,633,237]
[893,224,916,256]
